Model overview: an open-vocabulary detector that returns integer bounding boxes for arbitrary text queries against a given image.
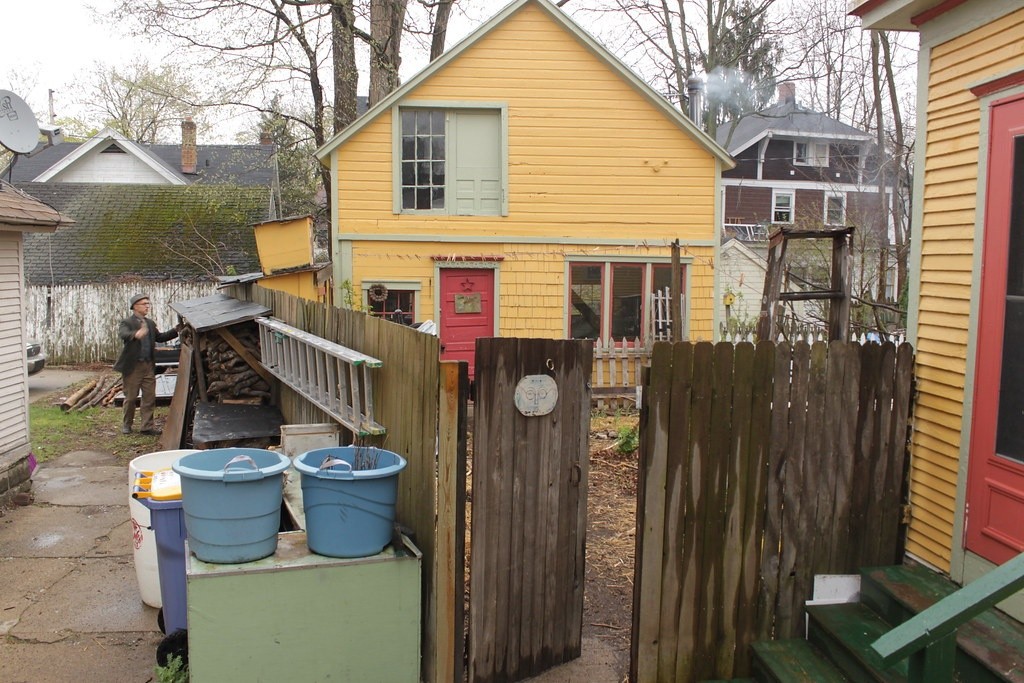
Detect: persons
[113,294,179,434]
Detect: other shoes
[123,426,131,434]
[140,429,162,435]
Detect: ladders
[252,316,386,437]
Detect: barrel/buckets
[292,446,407,558]
[129,449,203,608]
[172,447,291,564]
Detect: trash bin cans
[134,468,188,671]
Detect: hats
[130,294,149,310]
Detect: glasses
[135,302,149,305]
